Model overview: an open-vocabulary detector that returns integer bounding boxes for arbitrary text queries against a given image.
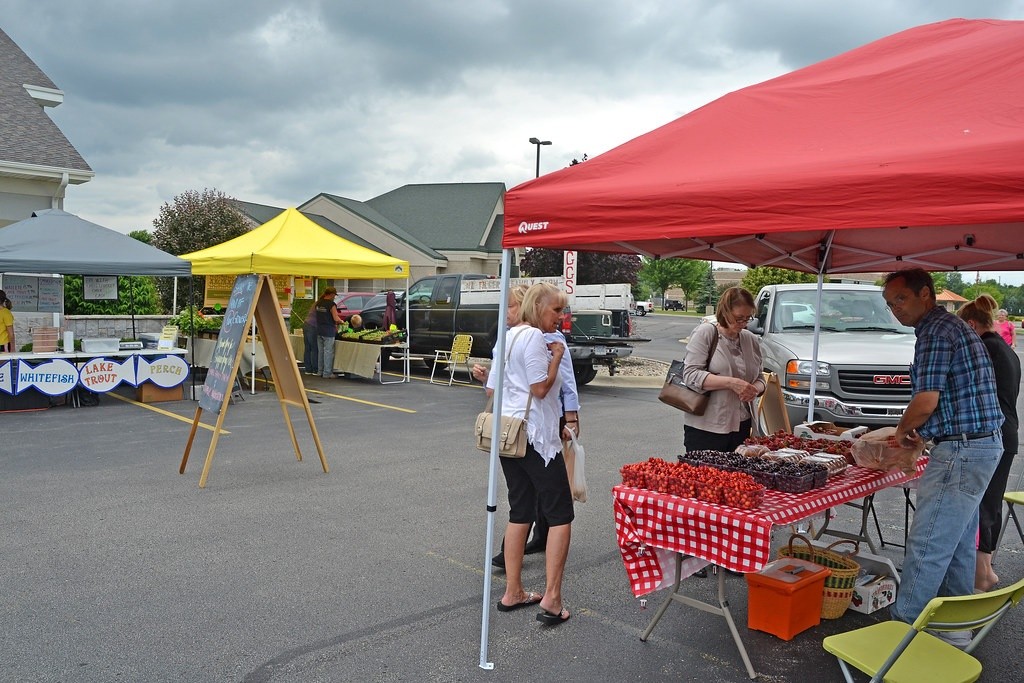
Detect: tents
[477,14,1024,668]
[177,206,410,384]
[0,209,195,402]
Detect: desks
[184,337,271,392]
[611,455,932,679]
[288,334,408,384]
[0,348,189,410]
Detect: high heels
[712,563,743,577]
[692,568,707,579]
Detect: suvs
[745,283,918,436]
[665,300,686,311]
[280,293,435,325]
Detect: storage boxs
[80,338,121,353]
[745,557,831,641]
[809,540,902,615]
[794,420,868,444]
[137,381,183,402]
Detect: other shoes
[317,372,322,376]
[305,371,313,374]
[929,629,973,646]
[313,372,317,375]
[492,554,504,568]
[323,373,338,378]
[524,540,542,555]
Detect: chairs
[824,579,1024,683]
[991,469,1024,564]
[430,335,474,386]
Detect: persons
[681,287,767,579]
[473,284,579,570]
[880,268,1004,650]
[950,293,1021,596]
[498,283,575,622]
[303,286,338,378]
[0,289,16,352]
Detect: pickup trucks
[345,273,652,385]
[630,301,653,317]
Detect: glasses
[727,310,755,325]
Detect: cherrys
[618,457,765,510]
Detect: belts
[934,432,993,441]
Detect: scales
[120,342,142,349]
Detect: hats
[325,286,339,296]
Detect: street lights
[529,138,552,178]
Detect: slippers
[498,591,543,612]
[973,588,985,593]
[535,608,571,623]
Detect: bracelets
[565,419,578,423]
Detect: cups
[294,329,303,335]
[376,363,380,373]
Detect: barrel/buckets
[338,322,349,333]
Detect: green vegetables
[165,305,225,337]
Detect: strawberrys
[745,427,857,465]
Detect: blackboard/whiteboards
[82,275,118,301]
[197,274,310,414]
[1,273,63,313]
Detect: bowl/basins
[619,462,766,509]
[677,450,828,493]
[842,449,853,464]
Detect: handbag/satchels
[560,409,579,439]
[778,535,861,620]
[561,425,590,504]
[659,323,719,417]
[474,412,528,458]
